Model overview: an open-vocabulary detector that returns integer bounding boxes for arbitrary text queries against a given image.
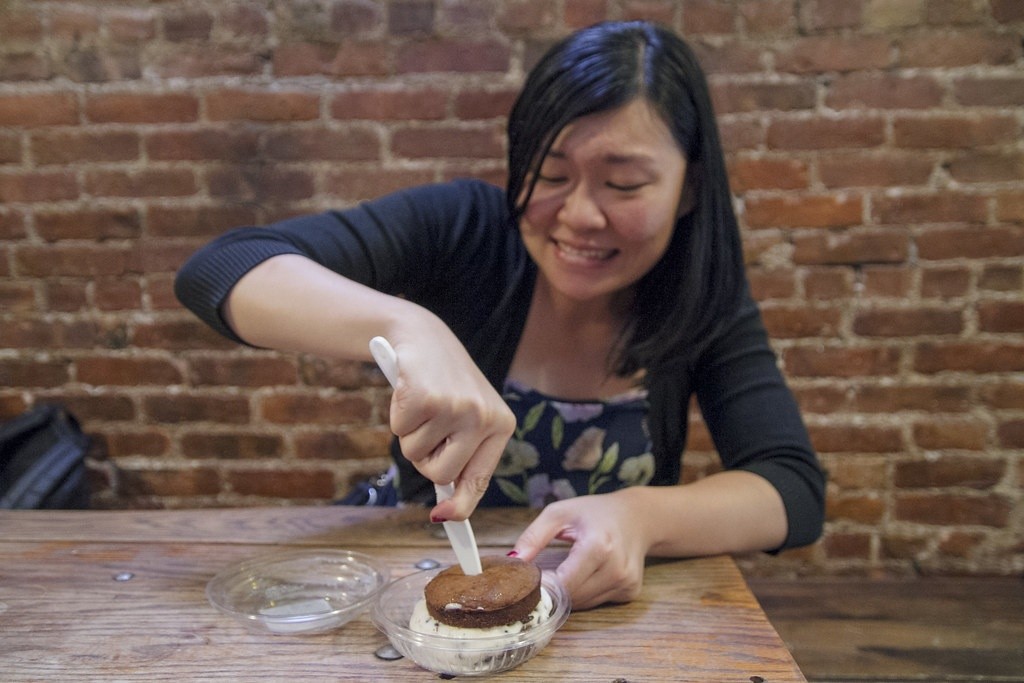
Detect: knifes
[368,337,483,576]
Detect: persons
[174,20,827,612]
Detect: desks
[0,503,809,683]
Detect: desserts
[410,588,553,674]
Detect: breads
[424,556,543,626]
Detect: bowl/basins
[372,567,570,676]
[205,548,389,635]
